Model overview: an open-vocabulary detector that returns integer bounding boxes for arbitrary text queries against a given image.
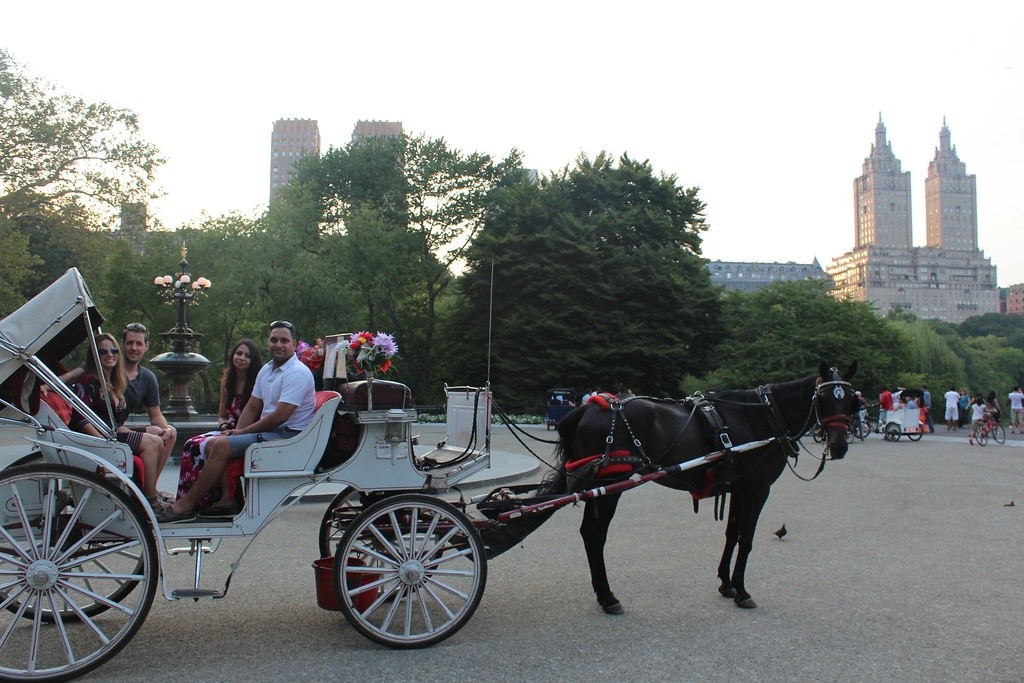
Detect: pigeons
[772,524,787,540]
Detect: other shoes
[155,490,176,504]
[1020,431,1024,434]
[929,429,934,433]
[150,497,171,513]
[969,439,973,445]
[1011,431,1015,433]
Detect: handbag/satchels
[983,415,987,423]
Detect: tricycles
[546,388,577,431]
[853,402,924,442]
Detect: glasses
[127,323,148,342]
[235,350,251,359]
[268,320,294,341]
[98,347,119,356]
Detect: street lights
[147,239,212,416]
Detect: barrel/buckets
[347,571,381,612]
[313,530,366,611]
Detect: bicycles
[975,409,1005,446]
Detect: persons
[921,386,935,433]
[944,388,971,431]
[549,394,576,406]
[148,321,316,523]
[581,388,601,405]
[894,395,920,411]
[969,391,1003,444]
[41,323,177,512]
[879,386,893,424]
[1005,386,1024,434]
[855,391,868,435]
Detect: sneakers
[198,503,241,517]
[147,504,196,524]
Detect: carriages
[0,265,869,683]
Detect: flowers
[296,338,324,370]
[339,330,398,371]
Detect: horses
[535,359,858,617]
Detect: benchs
[548,404,576,418]
[325,333,414,424]
[222,391,342,476]
[40,390,144,487]
